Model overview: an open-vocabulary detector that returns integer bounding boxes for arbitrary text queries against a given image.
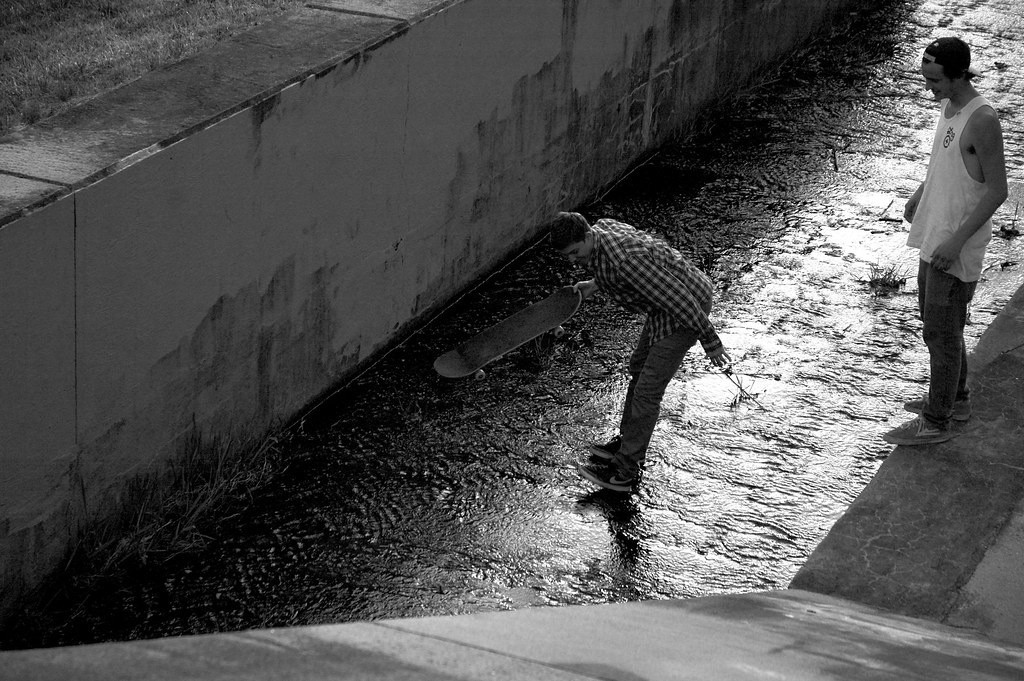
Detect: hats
[923,37,984,77]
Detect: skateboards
[434,283,583,381]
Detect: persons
[549,210,731,493]
[884,36,1007,445]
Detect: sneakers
[578,462,631,492]
[591,435,646,462]
[882,416,951,444]
[903,393,971,421]
